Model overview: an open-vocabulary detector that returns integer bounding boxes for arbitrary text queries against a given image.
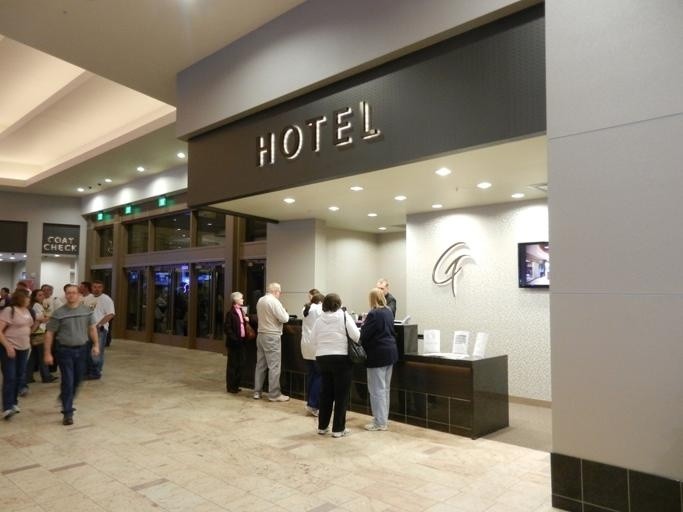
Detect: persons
[301,294,325,416]
[1,280,116,424]
[309,289,320,300]
[224,292,250,393]
[375,278,397,317]
[359,286,399,430]
[312,293,361,437]
[254,282,290,402]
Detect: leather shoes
[40,376,59,384]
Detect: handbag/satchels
[347,335,368,366]
[242,321,257,339]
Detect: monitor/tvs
[517,240,550,288]
[0,219,28,253]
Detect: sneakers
[253,391,263,400]
[227,387,242,394]
[268,393,291,403]
[1,404,21,422]
[303,404,320,418]
[62,417,73,426]
[331,428,352,438]
[316,425,332,435]
[362,423,390,432]
[19,384,29,396]
